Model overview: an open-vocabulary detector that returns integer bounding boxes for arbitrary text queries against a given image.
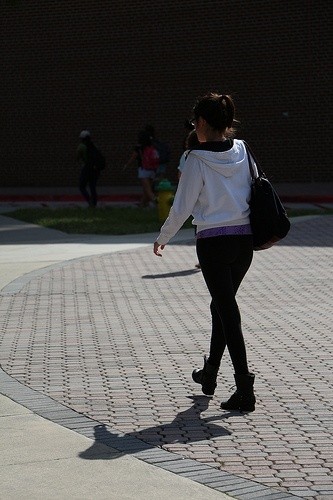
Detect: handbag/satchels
[242,139,290,251]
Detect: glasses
[189,117,199,126]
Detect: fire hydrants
[151,178,176,229]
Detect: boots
[220,372,256,412]
[191,355,221,395]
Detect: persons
[176,128,201,269]
[119,127,165,210]
[75,130,102,206]
[153,91,259,412]
